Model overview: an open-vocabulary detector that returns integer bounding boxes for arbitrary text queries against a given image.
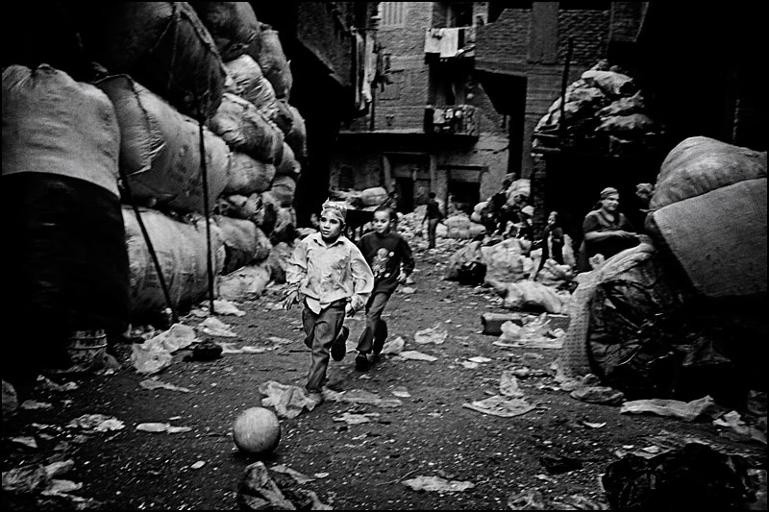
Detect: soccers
[233,407,279,454]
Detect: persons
[421,192,440,249]
[579,187,636,273]
[479,180,564,278]
[354,207,416,371]
[448,196,456,214]
[281,199,375,406]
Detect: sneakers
[331,326,349,362]
